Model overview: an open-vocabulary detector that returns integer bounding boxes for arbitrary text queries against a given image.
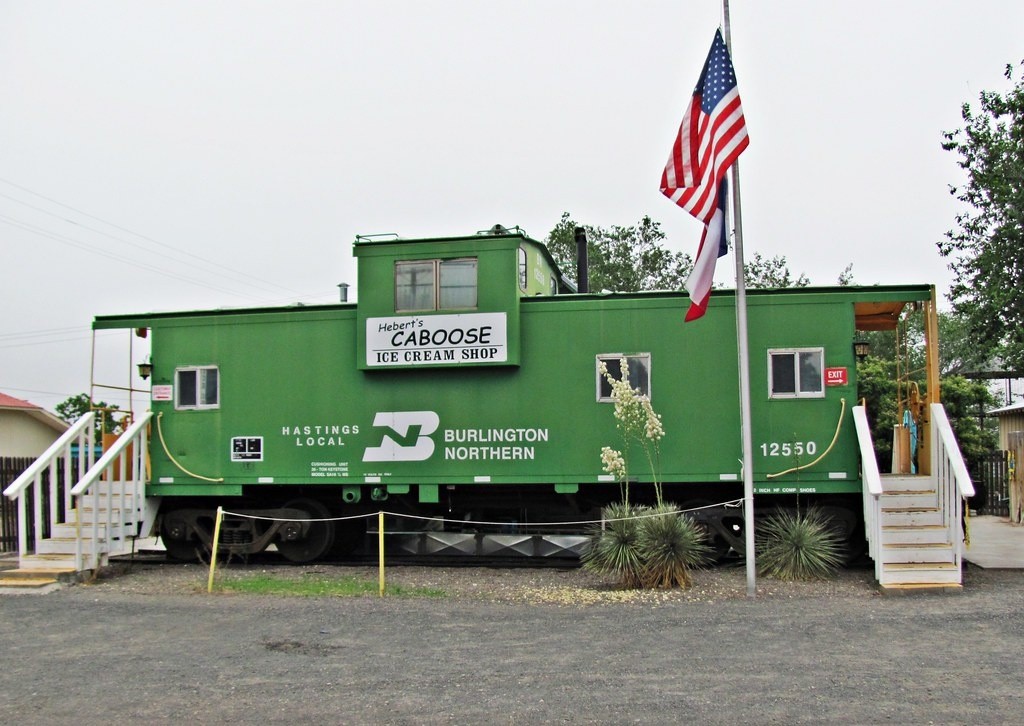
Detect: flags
[659,27,750,323]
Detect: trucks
[1,223,978,592]
